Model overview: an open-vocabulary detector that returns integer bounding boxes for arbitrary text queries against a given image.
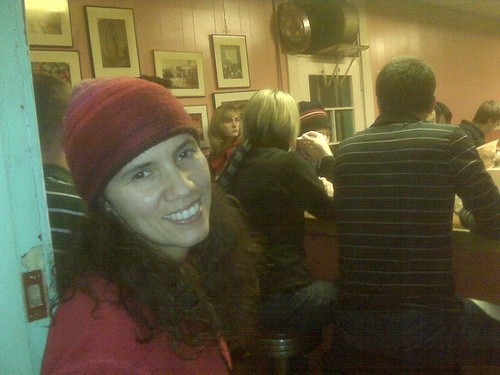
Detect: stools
[252,330,320,375]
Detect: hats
[297,101,332,136]
[60,74,203,216]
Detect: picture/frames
[211,33,251,89]
[29,48,82,91]
[184,104,210,149]
[151,49,206,98]
[23,0,73,47]
[213,88,260,109]
[84,5,141,81]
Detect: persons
[40,76,258,375]
[34,70,88,269]
[207,102,242,181]
[233,89,335,346]
[332,55,500,375]
[435,102,453,124]
[460,100,500,147]
[298,101,331,165]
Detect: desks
[304,211,500,306]
